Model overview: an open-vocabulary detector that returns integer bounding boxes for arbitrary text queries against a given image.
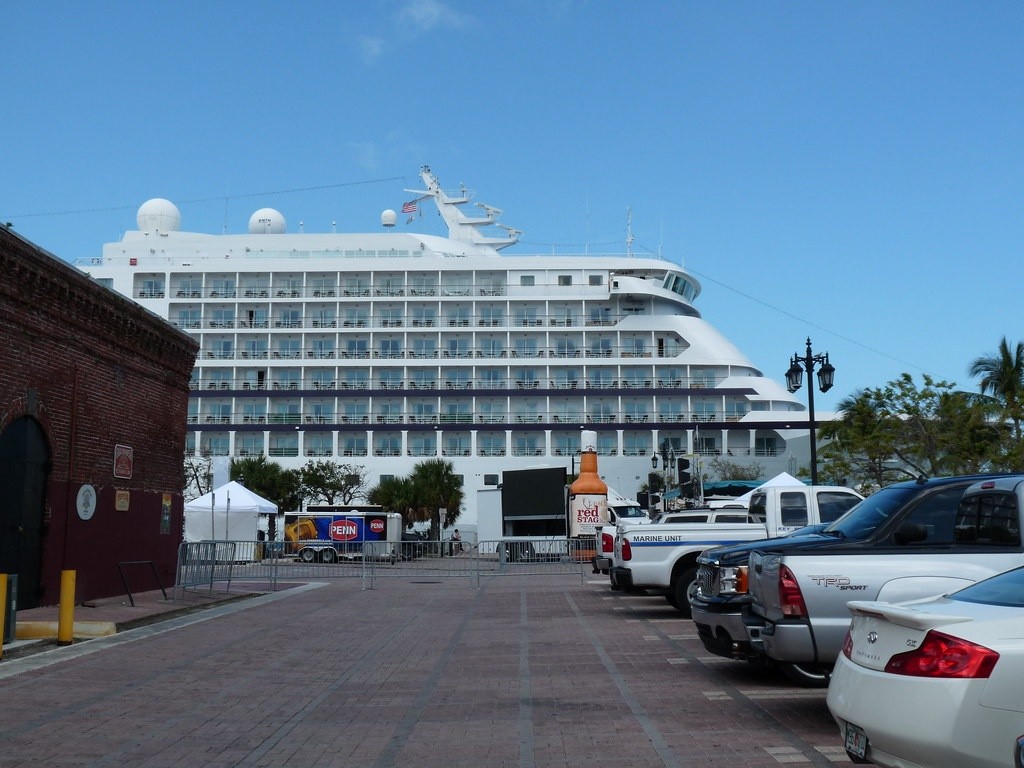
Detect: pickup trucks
[613,485,890,618]
[748,477,1024,681]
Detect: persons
[257,530,265,543]
[453,529,464,551]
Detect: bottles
[569,430,608,558]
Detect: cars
[830,564,1024,767]
[592,487,751,576]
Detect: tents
[735,472,807,508]
[185,481,279,562]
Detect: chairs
[139,289,751,456]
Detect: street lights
[784,336,835,484]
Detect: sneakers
[460,548,464,551]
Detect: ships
[73,165,807,564]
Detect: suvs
[690,474,1024,660]
[402,532,427,560]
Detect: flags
[401,200,416,212]
